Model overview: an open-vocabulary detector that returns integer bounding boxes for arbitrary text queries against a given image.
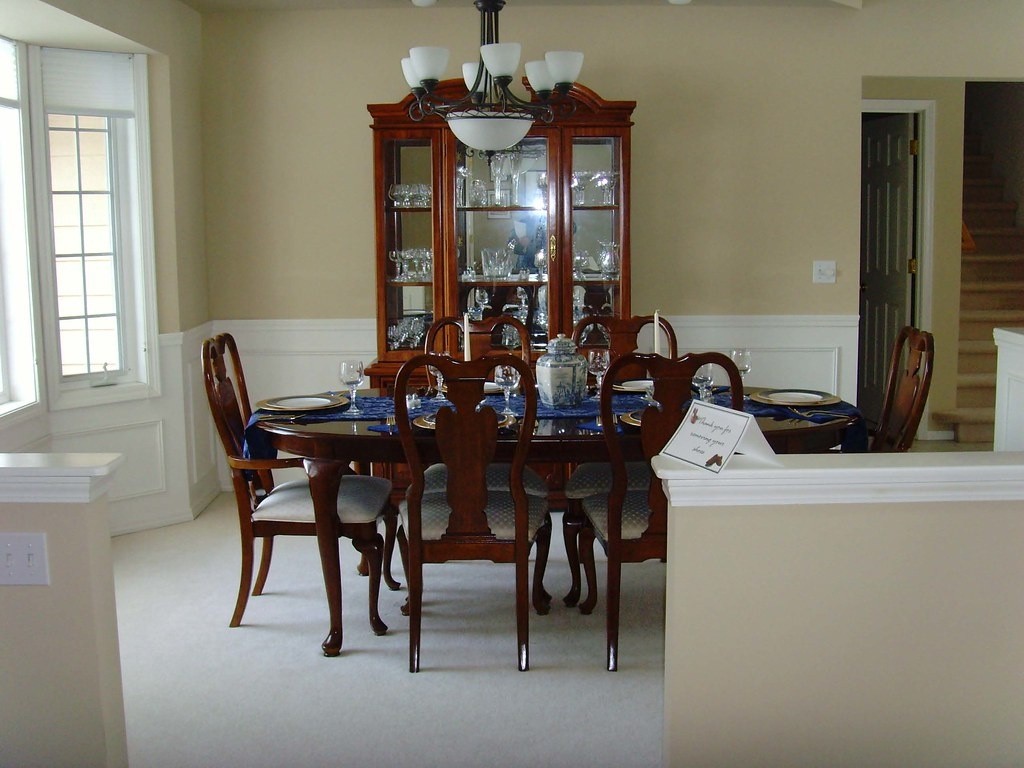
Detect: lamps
[484,145,544,173]
[400,1,584,151]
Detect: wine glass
[692,363,713,402]
[730,349,752,400]
[429,351,451,401]
[574,249,590,279]
[494,365,519,416]
[389,184,431,207]
[587,350,609,401]
[574,171,614,206]
[390,248,431,282]
[534,252,546,280]
[388,318,425,349]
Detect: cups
[469,179,486,207]
[481,249,512,280]
[502,324,520,349]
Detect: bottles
[406,395,414,409]
[340,361,364,414]
[601,245,619,278]
[412,394,421,408]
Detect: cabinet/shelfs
[366,75,637,387]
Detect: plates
[496,413,516,428]
[434,381,503,395]
[413,416,435,429]
[613,381,654,391]
[619,411,642,426]
[750,388,840,405]
[257,394,349,410]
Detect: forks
[386,416,395,435]
[787,407,849,417]
[596,416,604,432]
[613,414,617,430]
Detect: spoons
[258,414,306,420]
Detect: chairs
[200,316,933,671]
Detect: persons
[509,213,577,272]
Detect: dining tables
[255,380,861,654]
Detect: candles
[654,310,661,355]
[463,314,471,362]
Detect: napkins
[327,390,351,399]
[692,384,730,395]
[578,419,625,437]
[788,409,858,422]
[368,424,400,435]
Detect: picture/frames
[487,188,511,219]
[490,157,513,182]
